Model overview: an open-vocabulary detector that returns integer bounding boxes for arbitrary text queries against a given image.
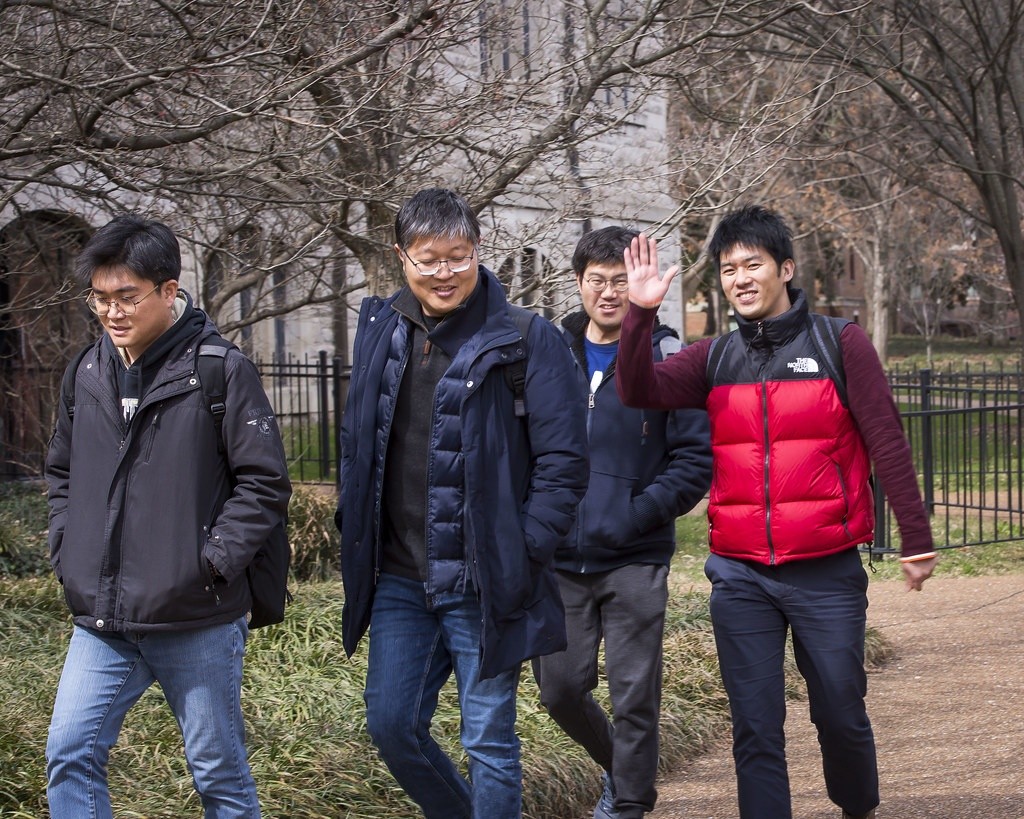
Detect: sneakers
[594,771,619,819]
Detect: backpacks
[63,332,290,627]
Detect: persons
[531,225,716,818]
[41,215,296,819]
[615,205,938,818]
[334,187,591,819]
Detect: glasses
[580,273,630,292]
[400,241,475,276]
[85,285,160,316]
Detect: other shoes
[842,808,875,818]
